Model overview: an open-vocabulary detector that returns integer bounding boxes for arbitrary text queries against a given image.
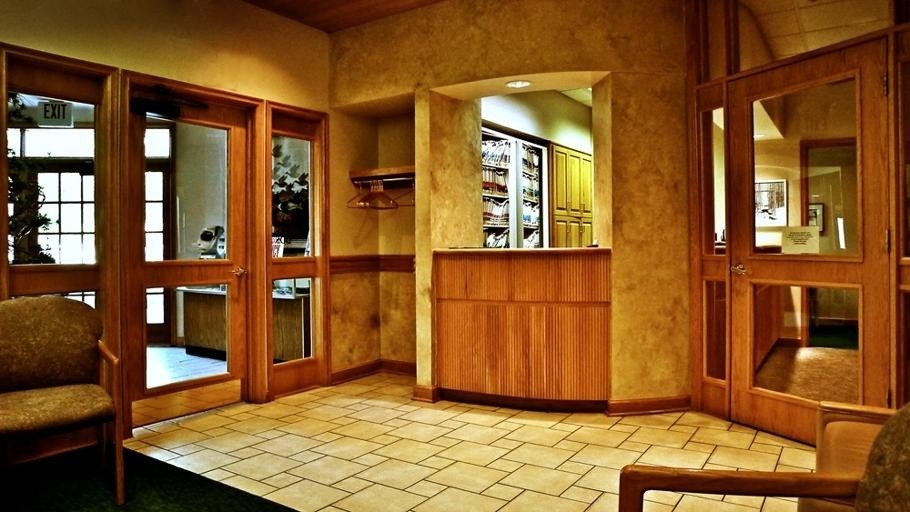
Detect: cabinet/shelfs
[482,126,549,248]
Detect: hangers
[346,177,415,209]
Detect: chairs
[617,402,909,512]
[0,295,126,505]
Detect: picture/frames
[755,179,788,232]
[808,202,824,232]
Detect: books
[479,138,541,249]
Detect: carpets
[0,442,300,512]
[808,323,858,349]
